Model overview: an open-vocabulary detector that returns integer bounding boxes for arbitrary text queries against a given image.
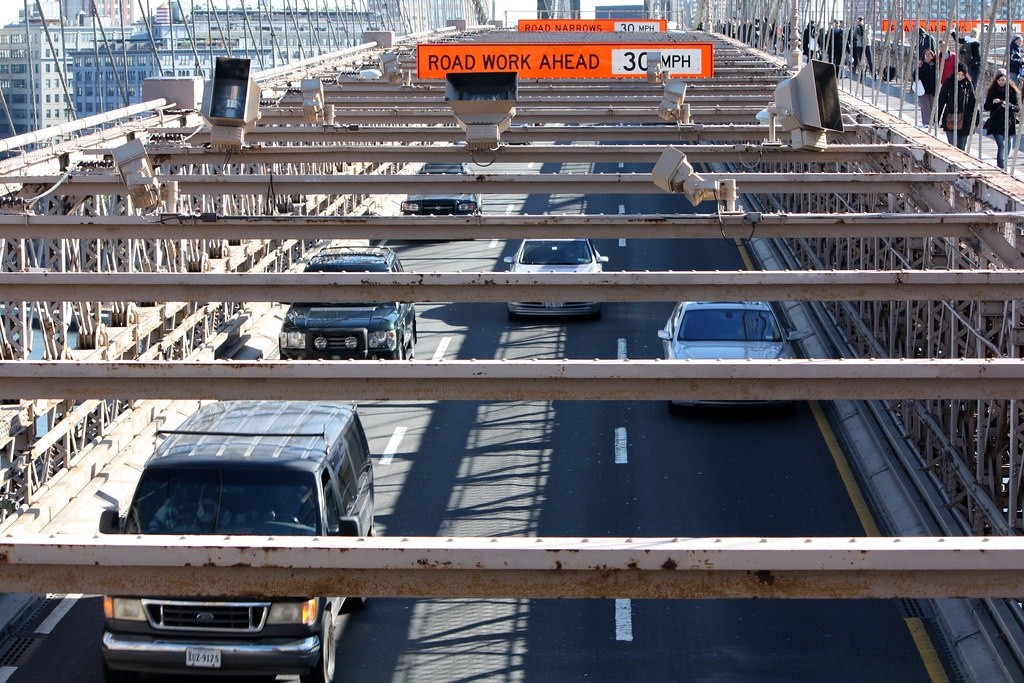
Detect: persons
[913,26,1023,172]
[713,15,877,83]
[148,480,216,533]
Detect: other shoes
[924,124,929,128]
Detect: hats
[994,68,1007,80]
[970,28,979,39]
[958,61,968,74]
[857,15,864,20]
[1011,34,1021,42]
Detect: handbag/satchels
[911,63,925,96]
[808,28,819,51]
[946,113,963,130]
[983,118,991,136]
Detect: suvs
[97,401,376,683]
[400,163,484,216]
[279,245,417,360]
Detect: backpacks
[958,42,974,66]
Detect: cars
[656,301,804,418]
[509,123,536,145]
[503,238,609,324]
